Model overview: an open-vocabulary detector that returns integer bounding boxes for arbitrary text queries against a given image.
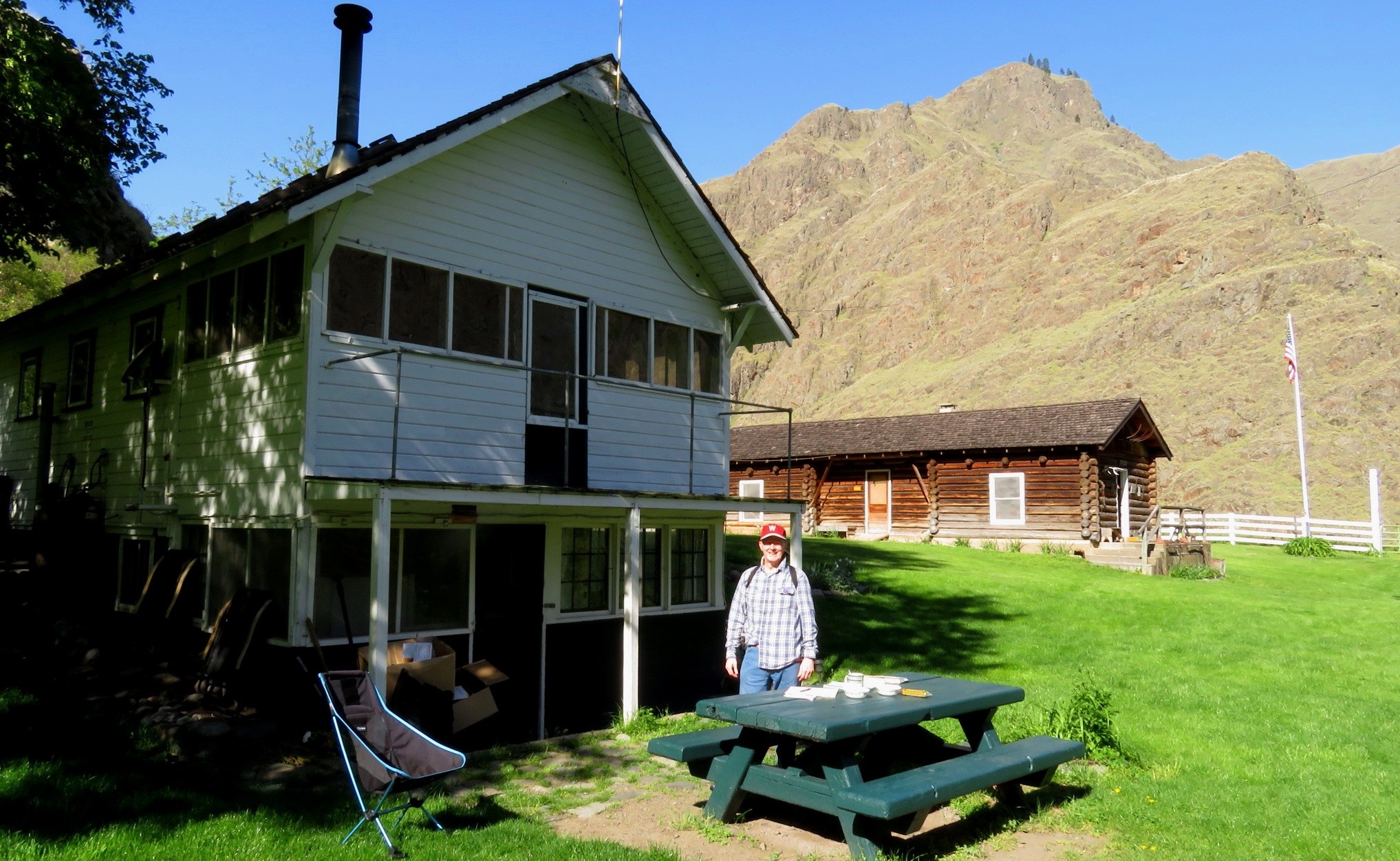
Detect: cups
[844,672,864,686]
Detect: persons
[724,525,819,770]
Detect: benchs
[647,726,749,762]
[816,525,859,540]
[834,739,1087,818]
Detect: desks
[698,669,1027,860]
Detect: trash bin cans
[358,636,456,750]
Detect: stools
[1099,527,1122,543]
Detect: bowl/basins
[845,686,870,699]
[879,684,901,696]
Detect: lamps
[451,505,477,524]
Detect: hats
[760,523,785,540]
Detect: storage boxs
[358,635,456,706]
[452,660,511,732]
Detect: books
[864,675,907,685]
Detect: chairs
[319,667,467,850]
